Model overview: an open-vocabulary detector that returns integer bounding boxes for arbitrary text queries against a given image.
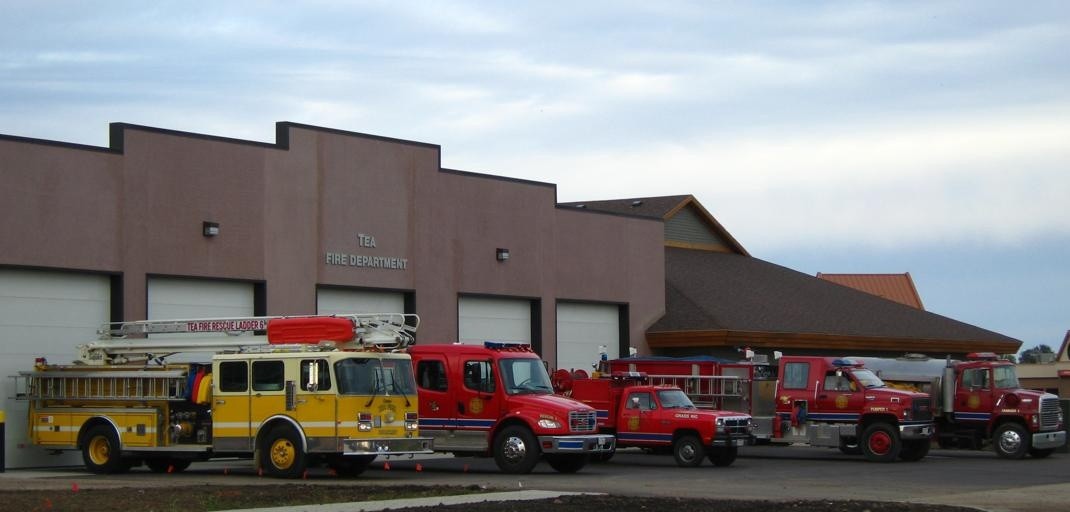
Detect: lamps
[203,221,220,237]
[496,248,509,261]
